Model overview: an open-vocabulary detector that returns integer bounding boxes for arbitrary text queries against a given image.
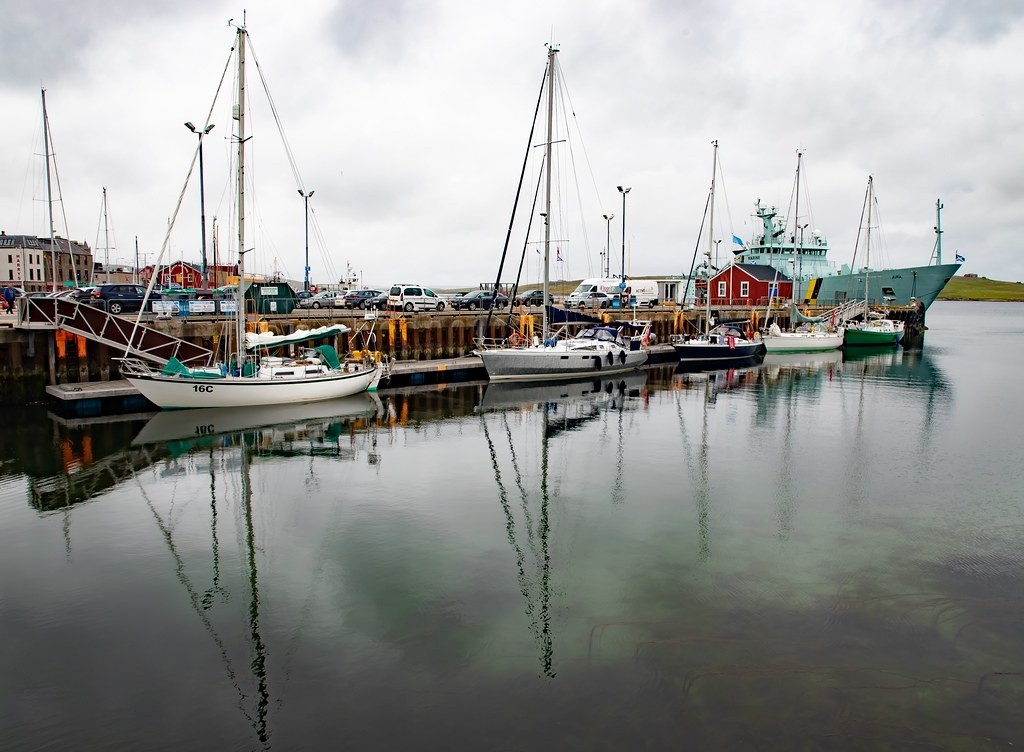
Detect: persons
[4,283,15,314]
[532,328,760,348]
[220,355,375,379]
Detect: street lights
[184,122,215,290]
[603,213,615,279]
[617,185,632,293]
[298,189,315,292]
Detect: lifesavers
[551,336,558,347]
[509,333,520,346]
[640,338,648,347]
[374,351,380,365]
[361,350,372,359]
[544,339,553,348]
[754,332,760,339]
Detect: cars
[563,292,610,309]
[299,290,341,309]
[294,291,313,303]
[333,289,351,309]
[0,284,93,311]
[513,290,554,306]
[451,290,509,311]
[365,290,393,311]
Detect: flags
[955,254,965,263]
[733,235,744,246]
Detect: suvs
[341,289,384,311]
[91,283,162,315]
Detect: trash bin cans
[179,293,190,317]
[771,296,785,309]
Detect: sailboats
[122,391,378,750]
[835,173,908,347]
[473,366,649,678]
[666,138,767,363]
[469,16,649,385]
[111,6,383,412]
[746,146,846,353]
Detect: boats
[691,196,963,313]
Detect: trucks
[571,277,660,311]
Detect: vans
[387,283,448,312]
